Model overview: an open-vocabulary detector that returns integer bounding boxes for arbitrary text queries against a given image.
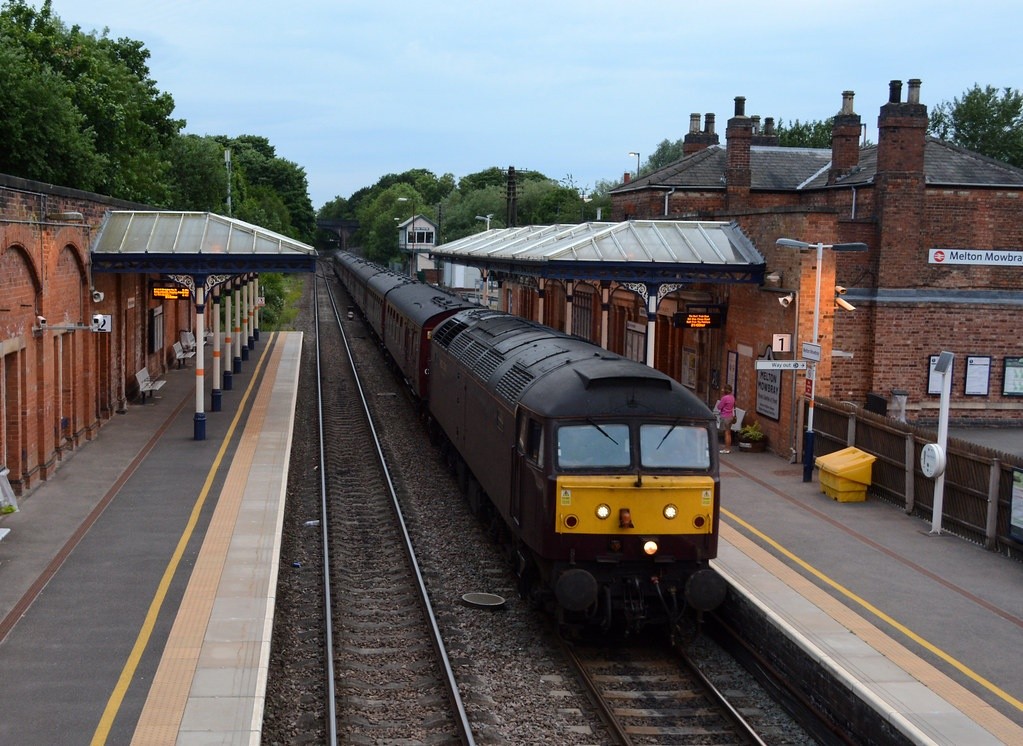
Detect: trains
[332,248,725,650]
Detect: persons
[716,384,736,454]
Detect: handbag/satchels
[732,416,737,424]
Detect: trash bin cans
[815,445,877,503]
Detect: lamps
[778,294,795,308]
[835,284,849,298]
[834,297,859,313]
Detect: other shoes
[719,449,732,454]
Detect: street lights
[475,213,494,231]
[398,197,415,276]
[629,152,640,179]
[775,237,868,483]
[224,149,231,219]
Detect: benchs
[173,340,196,367]
[185,331,207,351]
[135,365,167,401]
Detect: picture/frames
[964,355,991,396]
[1002,356,1023,397]
[926,354,954,396]
